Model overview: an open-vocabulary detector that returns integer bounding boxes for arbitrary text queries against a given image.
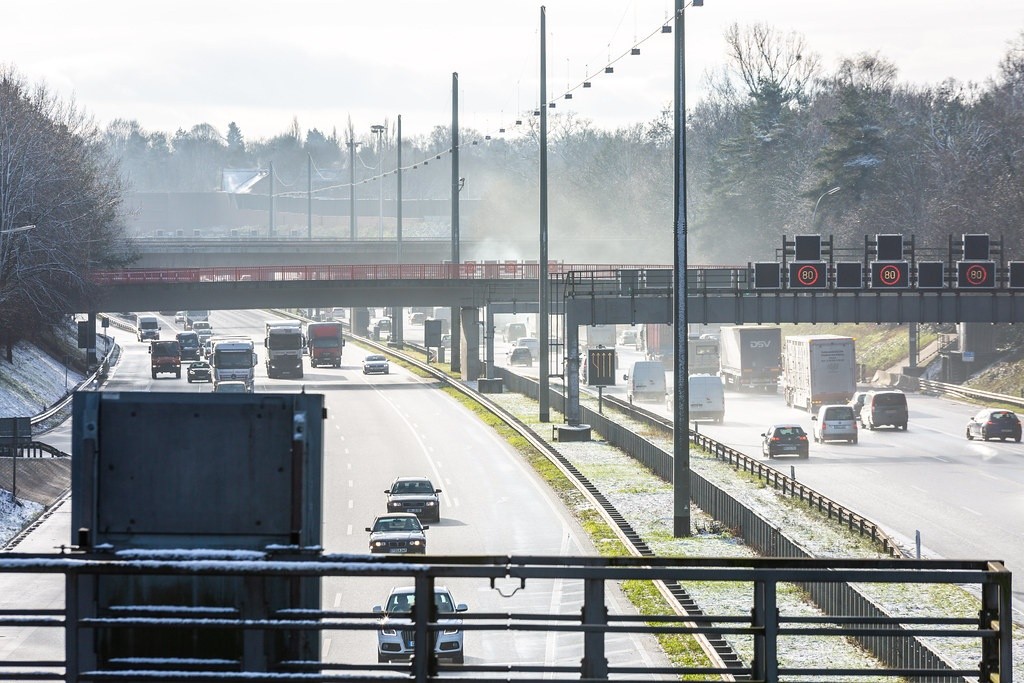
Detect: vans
[502,324,561,367]
[811,389,909,443]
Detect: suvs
[383,476,442,522]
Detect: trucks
[690,373,725,424]
[622,360,667,403]
[183,309,211,331]
[636,324,858,412]
[362,353,391,375]
[137,315,200,379]
[264,320,346,378]
[578,324,616,351]
[211,336,259,393]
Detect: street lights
[371,123,385,240]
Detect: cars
[967,408,1022,443]
[192,321,214,359]
[185,361,212,383]
[174,312,185,323]
[372,584,468,663]
[619,331,637,345]
[323,306,452,348]
[365,513,429,554]
[760,424,809,459]
